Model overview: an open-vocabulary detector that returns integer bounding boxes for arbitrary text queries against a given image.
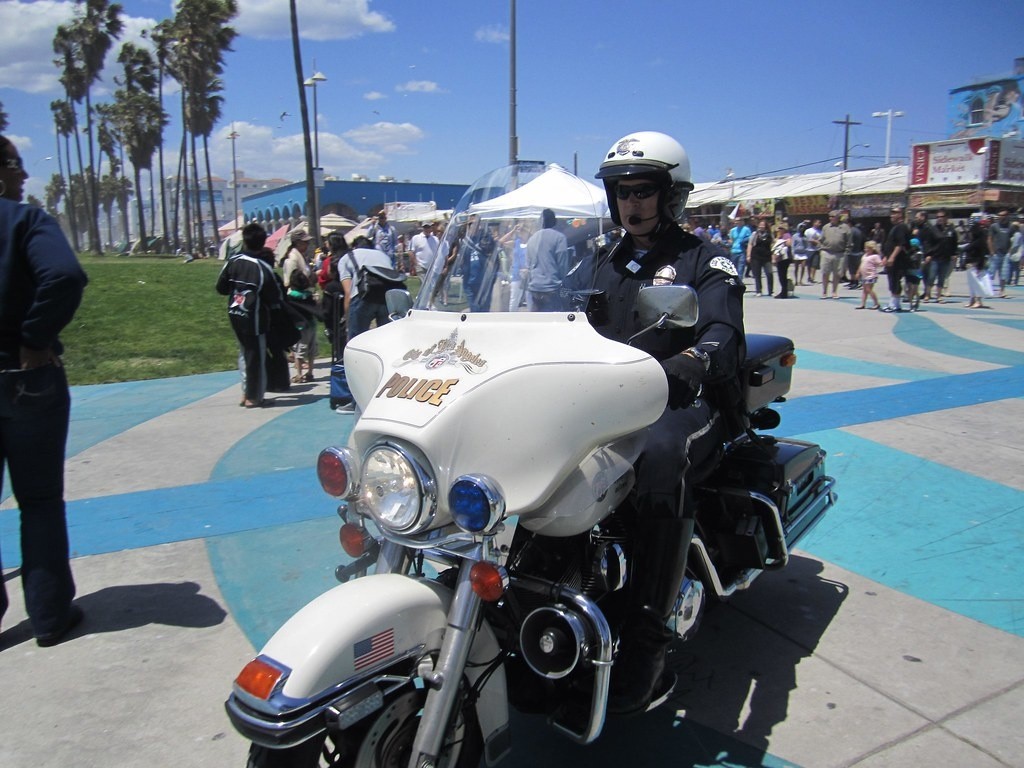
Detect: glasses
[613,183,661,200]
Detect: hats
[422,219,432,227]
[290,230,313,242]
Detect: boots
[606,520,694,720]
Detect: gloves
[660,353,706,410]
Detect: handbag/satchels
[354,265,408,304]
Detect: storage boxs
[743,333,796,414]
[725,435,826,541]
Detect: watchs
[685,348,710,371]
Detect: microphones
[628,210,664,225]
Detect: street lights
[225,120,242,233]
[871,108,904,167]
[303,56,329,248]
[844,142,871,171]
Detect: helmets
[594,131,694,222]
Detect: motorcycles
[221,161,839,768]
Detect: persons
[215,224,290,408]
[791,218,822,286]
[955,211,1024,309]
[0,137,87,649]
[283,230,348,382]
[409,209,568,310]
[539,130,747,714]
[681,216,791,299]
[819,210,852,299]
[176,248,194,264]
[842,204,958,314]
[337,236,408,342]
[369,211,396,268]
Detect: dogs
[287,352,311,377]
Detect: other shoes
[774,291,788,298]
[883,306,902,312]
[36,605,83,647]
[290,373,313,383]
[240,398,275,408]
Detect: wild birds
[280,111,291,121]
[166,175,173,180]
[43,156,53,161]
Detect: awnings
[687,164,909,208]
[398,210,454,223]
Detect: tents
[218,216,244,239]
[219,230,243,258]
[458,162,611,301]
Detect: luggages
[330,292,356,411]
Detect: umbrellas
[320,211,359,226]
